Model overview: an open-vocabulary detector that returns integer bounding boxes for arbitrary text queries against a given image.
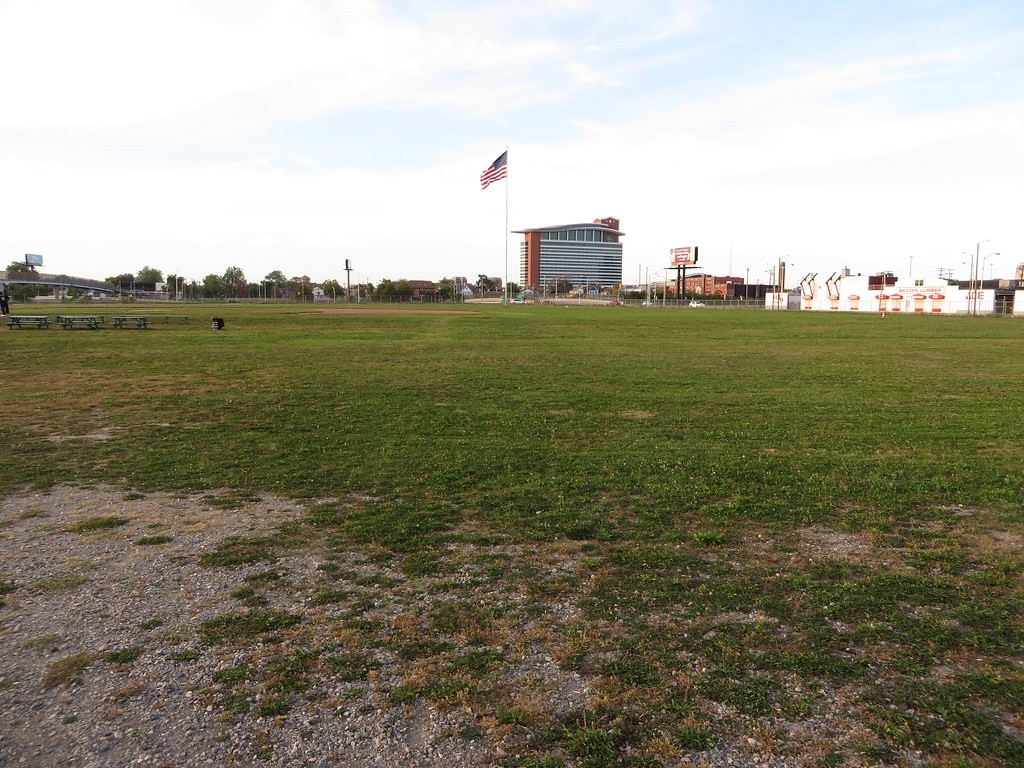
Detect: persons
[0,289,9,316]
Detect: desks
[63,315,99,330]
[112,316,148,329]
[9,315,49,330]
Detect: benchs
[56,321,104,330]
[6,321,52,330]
[153,316,190,324]
[110,322,153,330]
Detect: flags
[480,151,507,190]
